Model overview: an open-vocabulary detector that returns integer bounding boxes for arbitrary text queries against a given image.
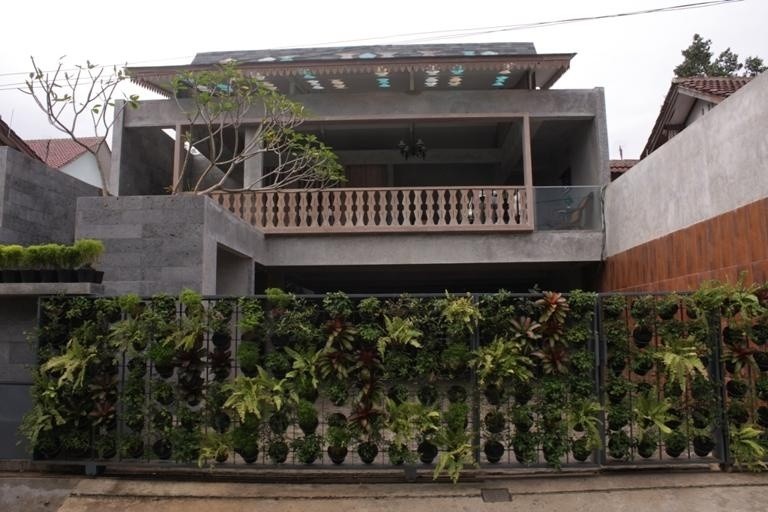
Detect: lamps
[398,121,428,161]
